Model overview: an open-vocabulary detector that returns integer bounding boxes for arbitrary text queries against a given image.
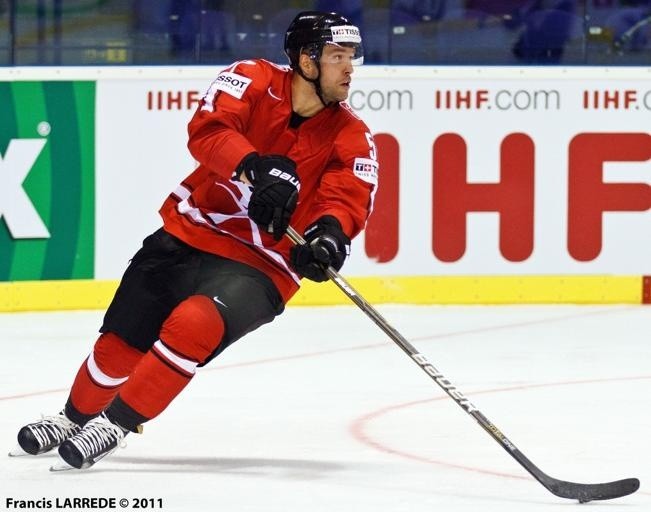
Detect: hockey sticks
[282,225,639,500]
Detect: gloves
[287,215,353,284]
[242,153,304,243]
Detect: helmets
[283,9,361,72]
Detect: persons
[16,8,383,472]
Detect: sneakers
[16,410,84,455]
[58,412,130,468]
